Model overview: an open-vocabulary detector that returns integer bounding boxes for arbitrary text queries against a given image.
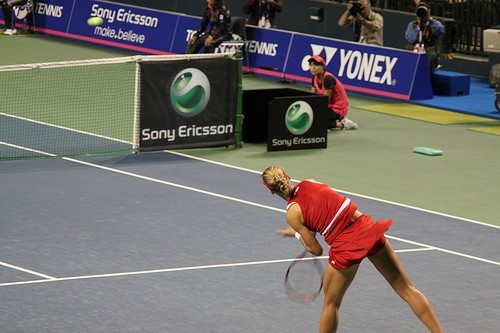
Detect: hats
[308,56,326,65]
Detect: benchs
[432,70,470,95]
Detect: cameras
[350,1,363,17]
[417,8,427,18]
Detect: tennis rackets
[283,227,323,304]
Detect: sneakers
[341,118,358,130]
[4,29,17,35]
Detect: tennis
[87,16,102,25]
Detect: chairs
[221,16,250,73]
[10,0,36,35]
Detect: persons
[308,56,358,130]
[262,166,444,333]
[243,0,282,27]
[185,0,231,54]
[406,3,445,74]
[0,0,28,36]
[338,0,383,46]
